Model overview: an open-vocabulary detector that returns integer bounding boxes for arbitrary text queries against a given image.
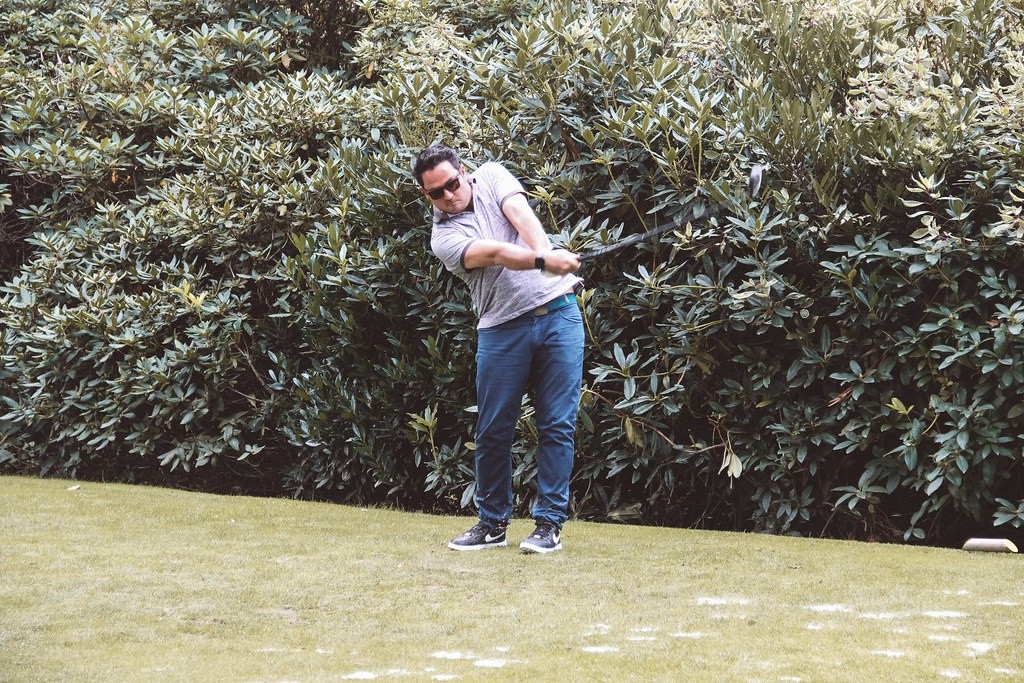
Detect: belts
[519,293,578,319]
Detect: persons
[412,148,584,552]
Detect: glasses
[426,176,461,200]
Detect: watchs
[535,253,545,269]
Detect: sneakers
[519,518,563,554]
[447,519,507,550]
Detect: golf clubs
[540,164,764,279]
